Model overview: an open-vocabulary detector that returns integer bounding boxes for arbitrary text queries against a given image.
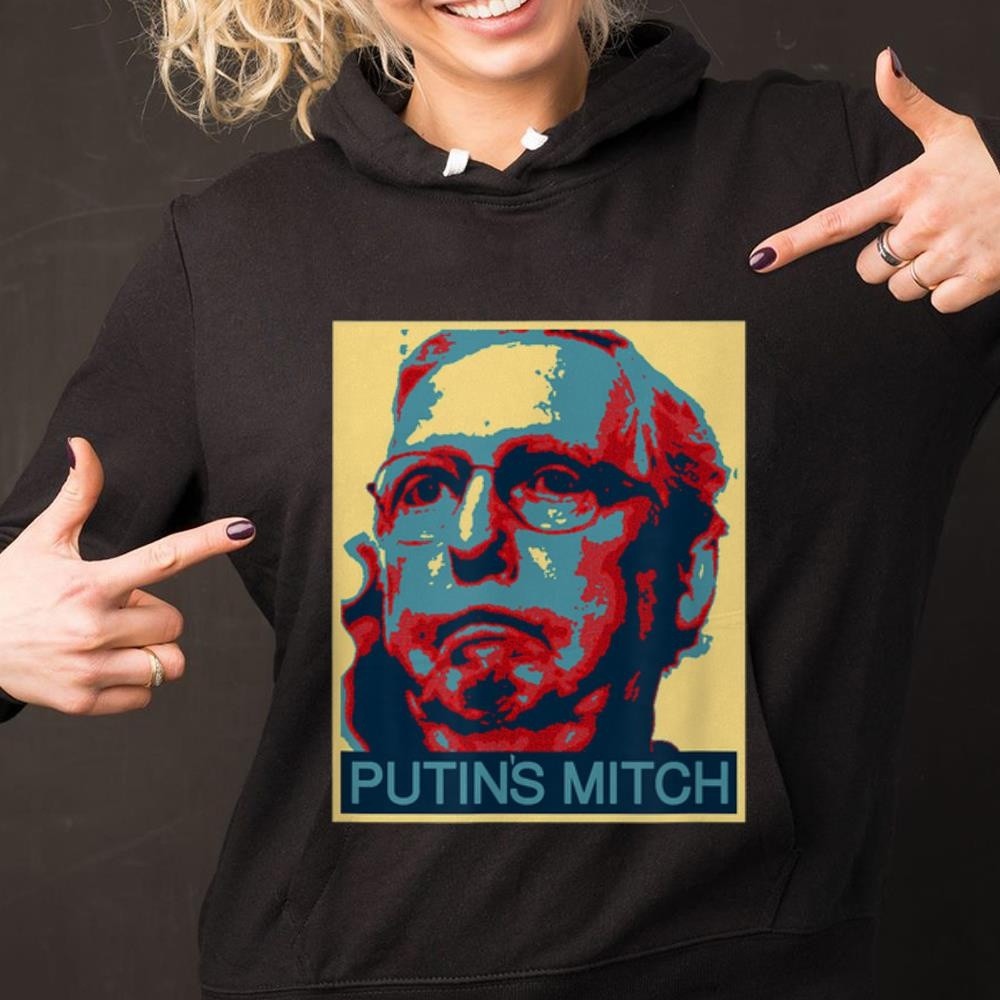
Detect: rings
[140,647,164,688]
[877,227,909,269]
[910,260,938,291]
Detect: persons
[0,0,1000,1000]
